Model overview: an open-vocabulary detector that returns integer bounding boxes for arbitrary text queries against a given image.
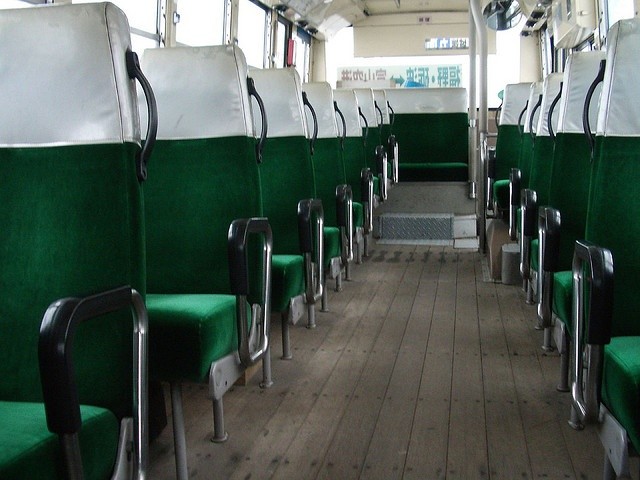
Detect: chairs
[0,0,145,480]
[297,81,343,284]
[245,66,329,329]
[371,88,398,187]
[331,89,375,242]
[543,51,612,345]
[568,18,640,480]
[515,82,554,242]
[485,81,534,211]
[352,88,390,211]
[134,44,274,444]
[528,72,567,280]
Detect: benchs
[387,87,473,182]
